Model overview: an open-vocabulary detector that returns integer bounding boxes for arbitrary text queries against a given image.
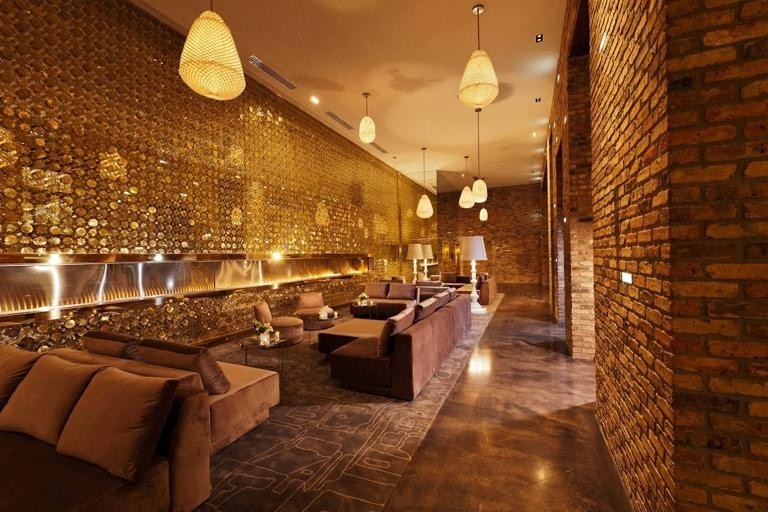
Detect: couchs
[1,343,212,510]
[46,332,279,455]
[318,274,499,402]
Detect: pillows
[1,343,43,408]
[1,354,105,446]
[81,333,145,363]
[136,339,231,395]
[56,367,180,482]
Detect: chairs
[253,302,304,346]
[294,292,334,331]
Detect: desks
[308,310,344,345]
[240,336,285,375]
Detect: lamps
[458,155,475,210]
[478,203,489,223]
[174,0,247,102]
[420,243,434,281]
[458,3,501,110]
[357,89,375,144]
[404,242,424,283]
[415,143,434,220]
[469,107,491,205]
[458,234,488,316]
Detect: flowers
[253,319,273,339]
